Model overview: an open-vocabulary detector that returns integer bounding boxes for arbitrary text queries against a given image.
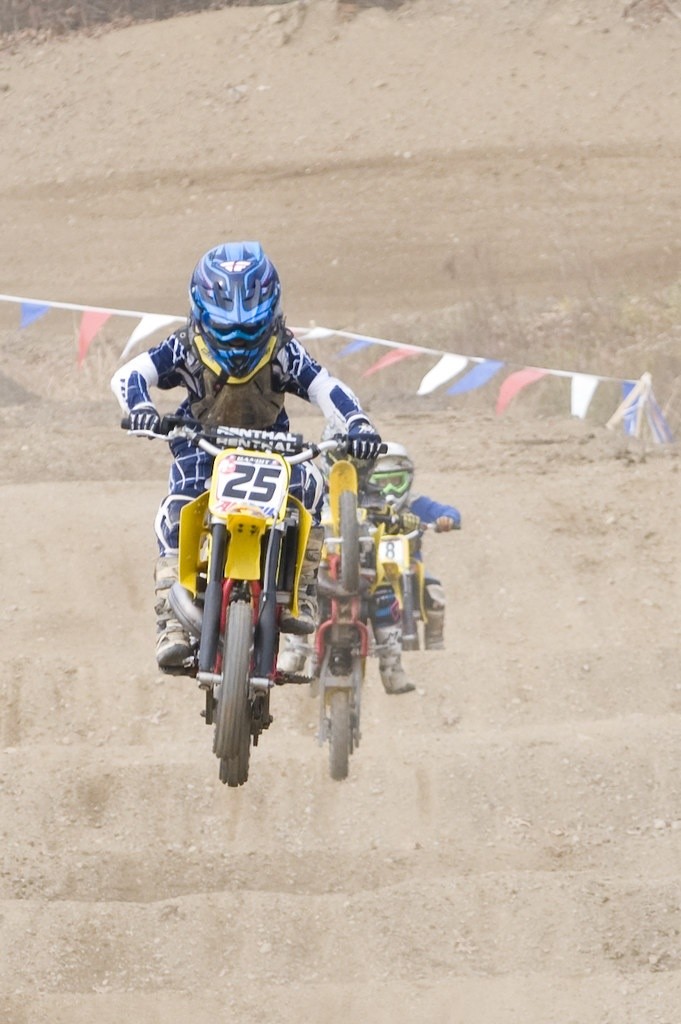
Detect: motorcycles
[119,413,389,788]
[300,460,459,781]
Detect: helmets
[365,442,415,513]
[187,240,282,379]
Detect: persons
[275,421,461,696]
[111,242,382,675]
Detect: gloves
[345,413,382,460]
[435,515,454,533]
[128,401,161,440]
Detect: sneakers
[279,595,316,635]
[276,641,309,674]
[153,617,194,668]
[379,666,417,695]
